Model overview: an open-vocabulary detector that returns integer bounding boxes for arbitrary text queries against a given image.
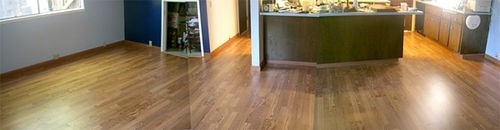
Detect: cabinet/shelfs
[414,1,492,58]
